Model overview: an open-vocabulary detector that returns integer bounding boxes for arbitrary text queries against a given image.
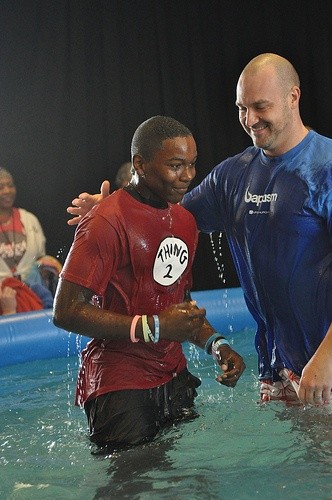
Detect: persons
[52,116,247,453]
[0,167,46,284]
[0,281,17,316]
[65,52,332,407]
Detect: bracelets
[204,333,230,360]
[145,315,159,344]
[140,315,151,342]
[129,315,142,343]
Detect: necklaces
[1,212,18,265]
